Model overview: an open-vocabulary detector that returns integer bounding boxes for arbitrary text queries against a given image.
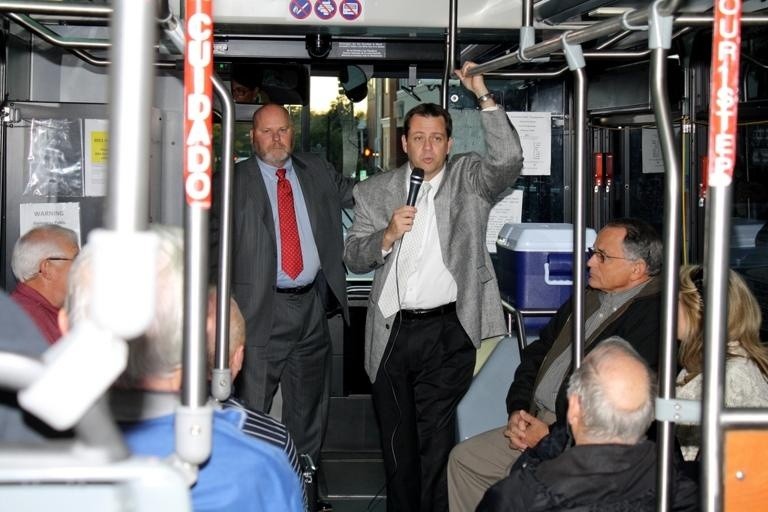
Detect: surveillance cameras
[304,29,333,58]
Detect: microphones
[403,168,425,204]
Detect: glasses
[587,247,626,263]
[688,268,704,308]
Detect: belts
[276,284,314,295]
[399,302,457,321]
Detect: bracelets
[479,91,494,103]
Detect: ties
[378,183,432,318]
[273,167,303,280]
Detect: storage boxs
[493,223,600,335]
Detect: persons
[447,222,767,511]
[231,77,269,106]
[345,60,523,511]
[211,102,361,442]
[122,224,306,511]
[1,224,80,447]
[209,282,310,511]
